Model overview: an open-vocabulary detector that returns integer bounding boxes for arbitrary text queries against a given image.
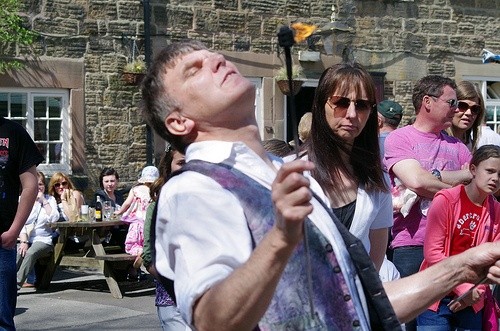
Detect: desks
[44,220,130,299]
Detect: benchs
[94,253,136,261]
[66,246,120,256]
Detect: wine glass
[103,201,111,221]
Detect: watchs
[431,168,442,181]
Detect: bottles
[95,196,102,222]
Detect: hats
[137,166,160,183]
[377,100,403,121]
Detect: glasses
[54,182,68,187]
[326,96,376,112]
[424,94,460,108]
[456,101,482,115]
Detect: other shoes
[22,282,34,287]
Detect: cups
[79,204,95,223]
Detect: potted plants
[124,55,147,84]
[274,63,305,95]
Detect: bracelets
[22,241,30,244]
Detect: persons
[47,172,87,249]
[0,116,45,331]
[15,172,59,290]
[141,39,500,331]
[280,60,393,276]
[91,166,131,245]
[140,146,193,331]
[263,75,500,331]
[113,166,160,267]
